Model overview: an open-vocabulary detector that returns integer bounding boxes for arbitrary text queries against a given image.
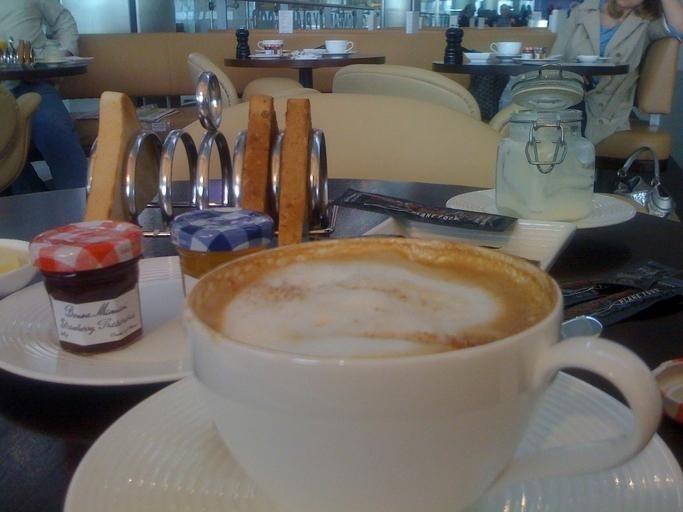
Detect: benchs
[53,26,680,171]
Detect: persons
[498,0,682,148]
[456,0,554,27]
[1,0,90,195]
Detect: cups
[179,235,663,510]
[489,40,522,55]
[324,39,353,52]
[257,38,283,55]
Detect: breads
[17,40,29,63]
[278,98,313,243]
[238,92,279,215]
[82,90,142,221]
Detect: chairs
[0,86,41,191]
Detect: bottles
[0,36,33,65]
[521,47,546,60]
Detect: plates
[1,250,178,392]
[61,374,679,512]
[321,51,353,57]
[595,55,612,63]
[352,215,574,269]
[443,189,638,229]
[248,54,282,58]
[512,57,560,65]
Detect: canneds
[29,221,144,357]
[169,206,274,296]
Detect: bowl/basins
[576,54,598,63]
[464,50,496,63]
[1,235,35,295]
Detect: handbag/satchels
[610,146,672,220]
